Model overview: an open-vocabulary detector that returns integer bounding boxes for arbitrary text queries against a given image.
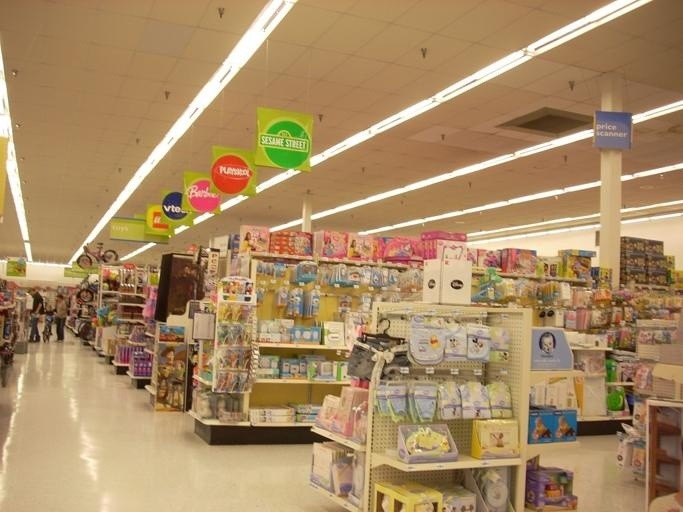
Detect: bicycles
[42,309,57,343]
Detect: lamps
[0,1,683,266]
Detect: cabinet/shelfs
[187,265,681,448]
[310,303,578,512]
[65,248,226,411]
[616,317,682,512]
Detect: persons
[26,288,44,342]
[53,294,66,343]
[555,416,575,438]
[39,302,56,335]
[539,332,555,353]
[534,417,551,438]
